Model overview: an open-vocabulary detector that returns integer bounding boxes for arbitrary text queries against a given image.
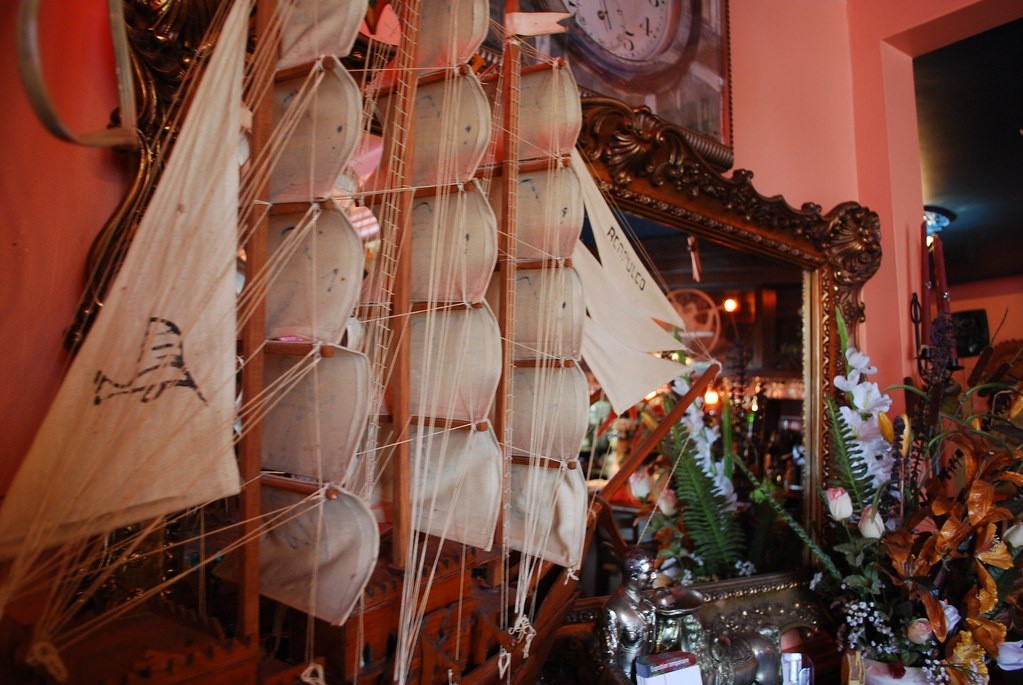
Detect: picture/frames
[470,0,735,173]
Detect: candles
[919,221,957,360]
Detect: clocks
[532,0,703,92]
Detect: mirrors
[521,92,885,657]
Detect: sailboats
[1,0,721,685]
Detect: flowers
[590,315,1023,685]
